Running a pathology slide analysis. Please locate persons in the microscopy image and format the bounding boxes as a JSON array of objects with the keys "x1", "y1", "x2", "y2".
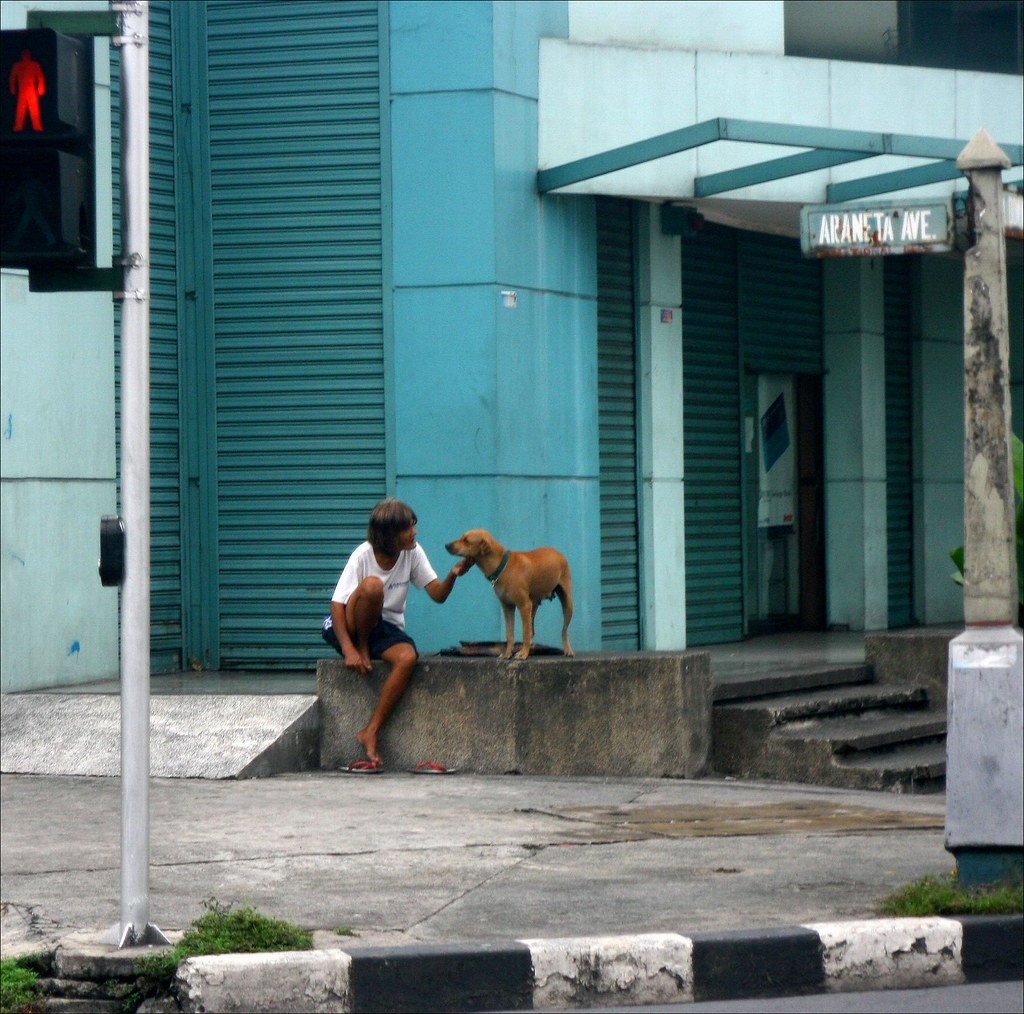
[{"x1": 322, "y1": 498, "x2": 475, "y2": 762}]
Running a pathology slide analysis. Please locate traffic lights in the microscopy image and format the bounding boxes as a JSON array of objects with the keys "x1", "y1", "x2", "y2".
[{"x1": 0, "y1": 25, "x2": 96, "y2": 268}]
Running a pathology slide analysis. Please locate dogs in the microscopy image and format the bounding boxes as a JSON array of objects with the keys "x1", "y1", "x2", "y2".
[{"x1": 445, "y1": 529, "x2": 574, "y2": 661}]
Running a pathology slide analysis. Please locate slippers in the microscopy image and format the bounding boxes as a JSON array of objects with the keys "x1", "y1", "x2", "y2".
[
  {"x1": 338, "y1": 761, "x2": 384, "y2": 773},
  {"x1": 407, "y1": 761, "x2": 456, "y2": 774}
]
